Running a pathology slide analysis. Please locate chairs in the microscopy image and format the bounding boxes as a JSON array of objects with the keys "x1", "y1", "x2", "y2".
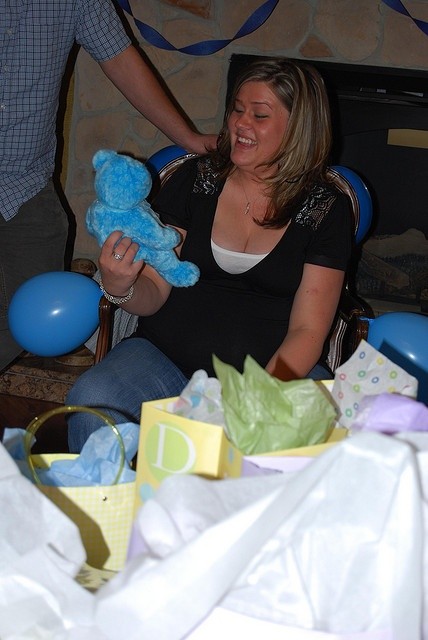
[{"x1": 93, "y1": 142, "x2": 371, "y2": 376}]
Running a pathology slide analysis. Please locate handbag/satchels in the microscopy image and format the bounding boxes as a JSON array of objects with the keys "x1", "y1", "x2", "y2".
[
  {"x1": 136, "y1": 377, "x2": 350, "y2": 512},
  {"x1": 13, "y1": 451, "x2": 138, "y2": 570}
]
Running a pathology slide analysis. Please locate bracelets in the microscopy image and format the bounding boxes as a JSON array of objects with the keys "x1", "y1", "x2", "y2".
[{"x1": 98, "y1": 277, "x2": 134, "y2": 305}]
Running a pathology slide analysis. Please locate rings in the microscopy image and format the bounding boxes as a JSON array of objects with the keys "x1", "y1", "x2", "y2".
[{"x1": 112, "y1": 251, "x2": 123, "y2": 261}]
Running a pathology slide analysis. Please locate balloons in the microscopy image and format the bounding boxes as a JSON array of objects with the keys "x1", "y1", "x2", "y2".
[
  {"x1": 358, "y1": 310, "x2": 428, "y2": 404},
  {"x1": 8, "y1": 271, "x2": 103, "y2": 357}
]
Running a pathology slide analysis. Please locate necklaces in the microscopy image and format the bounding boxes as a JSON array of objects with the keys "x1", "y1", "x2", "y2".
[{"x1": 237, "y1": 167, "x2": 272, "y2": 216}]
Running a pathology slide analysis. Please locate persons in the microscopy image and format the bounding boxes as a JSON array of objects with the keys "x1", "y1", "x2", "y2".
[
  {"x1": 0, "y1": 0, "x2": 220, "y2": 371},
  {"x1": 64, "y1": 59, "x2": 355, "y2": 454}
]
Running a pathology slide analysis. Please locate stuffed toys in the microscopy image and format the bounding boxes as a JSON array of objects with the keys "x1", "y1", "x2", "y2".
[{"x1": 85, "y1": 148, "x2": 201, "y2": 288}]
